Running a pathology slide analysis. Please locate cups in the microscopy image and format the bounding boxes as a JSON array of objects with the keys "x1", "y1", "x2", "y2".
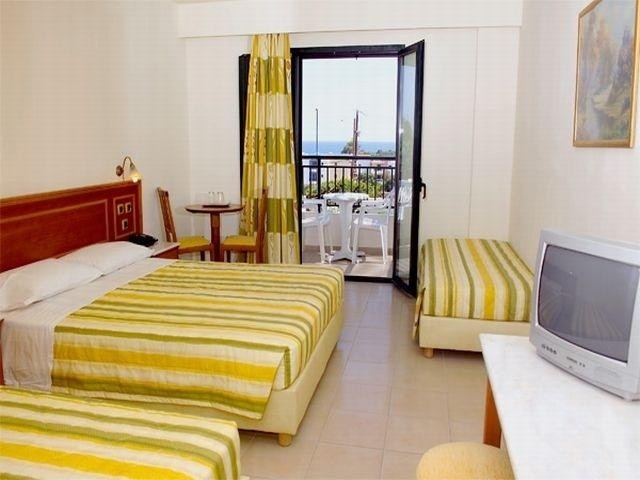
[
  {"x1": 216, "y1": 191, "x2": 224, "y2": 205},
  {"x1": 208, "y1": 191, "x2": 216, "y2": 204}
]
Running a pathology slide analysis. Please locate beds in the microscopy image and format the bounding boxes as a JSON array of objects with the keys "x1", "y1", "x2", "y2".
[
  {"x1": 0, "y1": 386, "x2": 250, "y2": 480},
  {"x1": 0, "y1": 182, "x2": 346, "y2": 445}
]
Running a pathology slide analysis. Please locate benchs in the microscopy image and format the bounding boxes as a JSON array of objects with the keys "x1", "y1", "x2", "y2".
[{"x1": 411, "y1": 237, "x2": 535, "y2": 359}]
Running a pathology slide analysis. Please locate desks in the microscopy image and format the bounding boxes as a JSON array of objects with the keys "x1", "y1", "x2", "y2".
[
  {"x1": 186, "y1": 204, "x2": 243, "y2": 261},
  {"x1": 322, "y1": 192, "x2": 369, "y2": 264},
  {"x1": 479, "y1": 334, "x2": 640, "y2": 480}
]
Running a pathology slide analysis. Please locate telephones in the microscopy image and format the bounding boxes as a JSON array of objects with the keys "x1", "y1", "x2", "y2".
[{"x1": 128, "y1": 233, "x2": 158, "y2": 247}]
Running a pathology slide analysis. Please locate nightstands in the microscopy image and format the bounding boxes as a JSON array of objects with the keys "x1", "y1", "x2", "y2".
[{"x1": 127, "y1": 234, "x2": 180, "y2": 259}]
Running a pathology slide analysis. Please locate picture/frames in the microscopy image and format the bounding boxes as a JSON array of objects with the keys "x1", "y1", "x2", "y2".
[{"x1": 573, "y1": 0, "x2": 640, "y2": 147}]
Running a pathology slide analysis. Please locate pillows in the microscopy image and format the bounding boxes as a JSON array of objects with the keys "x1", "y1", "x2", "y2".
[
  {"x1": 58, "y1": 241, "x2": 155, "y2": 276},
  {"x1": 0, "y1": 258, "x2": 104, "y2": 310}
]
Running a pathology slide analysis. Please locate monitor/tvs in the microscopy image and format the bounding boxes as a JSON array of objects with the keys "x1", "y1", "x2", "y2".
[{"x1": 529, "y1": 230, "x2": 640, "y2": 403}]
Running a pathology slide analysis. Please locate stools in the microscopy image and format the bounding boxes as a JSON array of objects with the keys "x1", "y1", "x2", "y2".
[{"x1": 416, "y1": 441, "x2": 514, "y2": 480}]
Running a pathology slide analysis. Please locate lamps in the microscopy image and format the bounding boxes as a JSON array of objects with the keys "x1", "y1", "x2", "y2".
[{"x1": 116, "y1": 156, "x2": 142, "y2": 184}]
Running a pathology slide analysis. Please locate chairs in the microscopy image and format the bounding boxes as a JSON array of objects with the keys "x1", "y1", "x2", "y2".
[
  {"x1": 299, "y1": 192, "x2": 333, "y2": 263},
  {"x1": 154, "y1": 187, "x2": 215, "y2": 261},
  {"x1": 351, "y1": 188, "x2": 395, "y2": 264},
  {"x1": 220, "y1": 189, "x2": 267, "y2": 262}
]
